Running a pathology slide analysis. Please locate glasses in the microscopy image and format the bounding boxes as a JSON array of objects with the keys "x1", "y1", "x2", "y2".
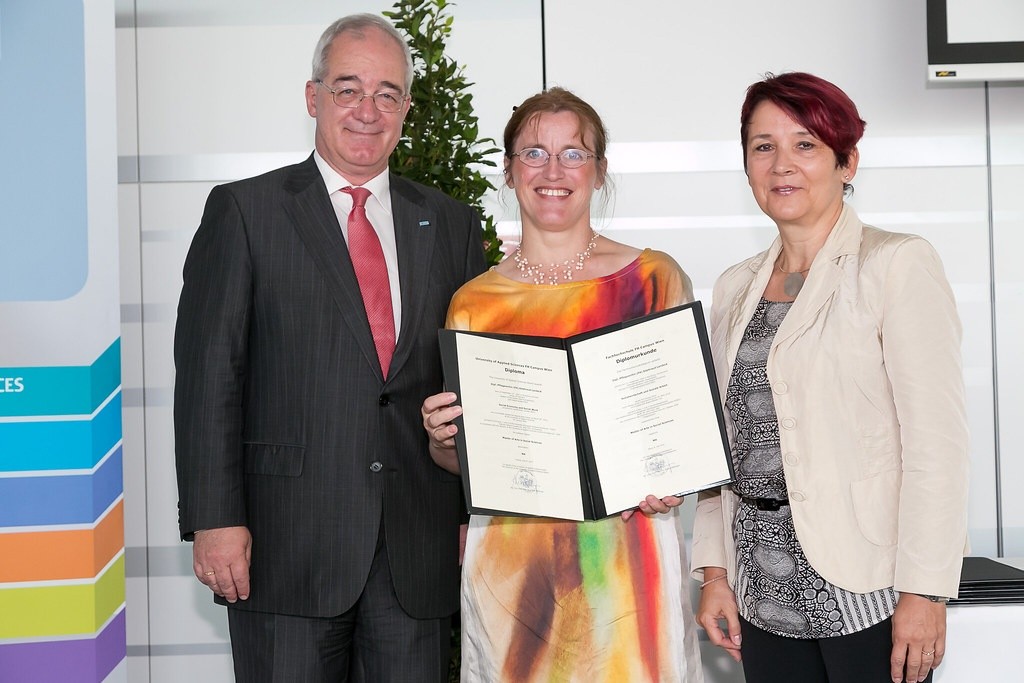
[
  {"x1": 509, "y1": 148, "x2": 599, "y2": 169},
  {"x1": 315, "y1": 79, "x2": 409, "y2": 113}
]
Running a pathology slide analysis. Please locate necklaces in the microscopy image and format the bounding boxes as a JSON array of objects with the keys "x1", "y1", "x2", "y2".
[
  {"x1": 777, "y1": 259, "x2": 810, "y2": 274},
  {"x1": 514, "y1": 228, "x2": 600, "y2": 285}
]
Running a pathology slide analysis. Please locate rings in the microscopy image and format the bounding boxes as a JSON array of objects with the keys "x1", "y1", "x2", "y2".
[
  {"x1": 203, "y1": 571, "x2": 215, "y2": 576},
  {"x1": 921, "y1": 649, "x2": 935, "y2": 656}
]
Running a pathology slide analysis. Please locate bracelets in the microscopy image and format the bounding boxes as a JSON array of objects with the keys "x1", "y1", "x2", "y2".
[{"x1": 699, "y1": 573, "x2": 727, "y2": 590}]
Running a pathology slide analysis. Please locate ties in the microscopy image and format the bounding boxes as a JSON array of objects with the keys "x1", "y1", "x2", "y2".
[{"x1": 338, "y1": 186, "x2": 396, "y2": 382}]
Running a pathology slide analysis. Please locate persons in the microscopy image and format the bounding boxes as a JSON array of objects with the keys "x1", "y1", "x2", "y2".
[
  {"x1": 690, "y1": 72, "x2": 970, "y2": 683},
  {"x1": 422, "y1": 87, "x2": 705, "y2": 683},
  {"x1": 173, "y1": 12, "x2": 489, "y2": 683}
]
